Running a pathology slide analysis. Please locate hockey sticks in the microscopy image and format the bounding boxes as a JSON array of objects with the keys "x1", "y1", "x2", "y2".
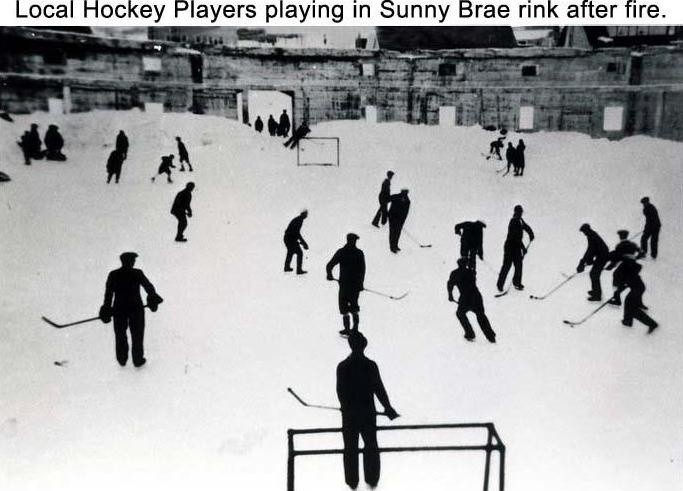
[
  {"x1": 40, "y1": 303, "x2": 149, "y2": 329},
  {"x1": 492, "y1": 241, "x2": 531, "y2": 296},
  {"x1": 529, "y1": 261, "x2": 588, "y2": 300},
  {"x1": 564, "y1": 284, "x2": 629, "y2": 326},
  {"x1": 333, "y1": 277, "x2": 409, "y2": 301},
  {"x1": 402, "y1": 226, "x2": 432, "y2": 249},
  {"x1": 286, "y1": 388, "x2": 386, "y2": 416}
]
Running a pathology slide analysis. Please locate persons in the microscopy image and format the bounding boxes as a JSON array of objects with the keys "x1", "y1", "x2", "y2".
[
  {"x1": 385, "y1": 189, "x2": 410, "y2": 252},
  {"x1": 448, "y1": 255, "x2": 496, "y2": 342},
  {"x1": 19, "y1": 122, "x2": 196, "y2": 241},
  {"x1": 336, "y1": 331, "x2": 398, "y2": 489},
  {"x1": 455, "y1": 221, "x2": 486, "y2": 273},
  {"x1": 100, "y1": 252, "x2": 163, "y2": 365},
  {"x1": 327, "y1": 234, "x2": 365, "y2": 336},
  {"x1": 497, "y1": 205, "x2": 534, "y2": 292},
  {"x1": 256, "y1": 110, "x2": 312, "y2": 148},
  {"x1": 578, "y1": 197, "x2": 660, "y2": 333},
  {"x1": 372, "y1": 171, "x2": 395, "y2": 226},
  {"x1": 487, "y1": 136, "x2": 527, "y2": 176},
  {"x1": 284, "y1": 210, "x2": 308, "y2": 274}
]
